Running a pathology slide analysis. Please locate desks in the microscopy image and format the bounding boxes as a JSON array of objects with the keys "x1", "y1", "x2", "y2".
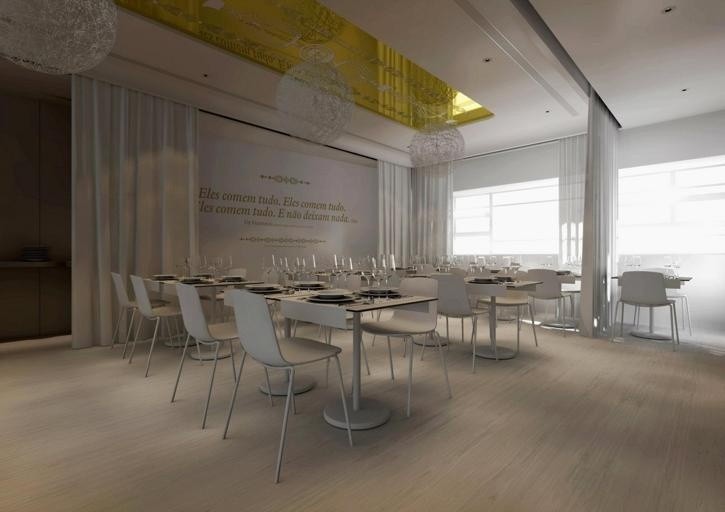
[
  {"x1": 611, "y1": 275, "x2": 691, "y2": 341},
  {"x1": 257, "y1": 290, "x2": 439, "y2": 431}
]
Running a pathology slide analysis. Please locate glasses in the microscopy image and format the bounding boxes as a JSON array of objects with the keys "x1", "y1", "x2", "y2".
[
  {"x1": 0, "y1": 0, "x2": 117, "y2": 75},
  {"x1": 276, "y1": 23, "x2": 354, "y2": 146},
  {"x1": 409, "y1": 106, "x2": 466, "y2": 170}
]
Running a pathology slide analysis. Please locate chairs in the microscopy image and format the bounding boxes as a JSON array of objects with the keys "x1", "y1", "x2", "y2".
[
  {"x1": 656, "y1": 270, "x2": 691, "y2": 332},
  {"x1": 107, "y1": 255, "x2": 575, "y2": 375},
  {"x1": 610, "y1": 271, "x2": 681, "y2": 351},
  {"x1": 360, "y1": 277, "x2": 453, "y2": 418},
  {"x1": 170, "y1": 282, "x2": 274, "y2": 429},
  {"x1": 222, "y1": 289, "x2": 354, "y2": 480},
  {"x1": 614, "y1": 272, "x2": 643, "y2": 330}
]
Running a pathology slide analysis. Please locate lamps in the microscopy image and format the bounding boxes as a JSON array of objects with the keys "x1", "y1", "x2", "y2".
[
  {"x1": 276, "y1": 23, "x2": 354, "y2": 146},
  {"x1": 0, "y1": 0, "x2": 117, "y2": 75},
  {"x1": 409, "y1": 106, "x2": 466, "y2": 170}
]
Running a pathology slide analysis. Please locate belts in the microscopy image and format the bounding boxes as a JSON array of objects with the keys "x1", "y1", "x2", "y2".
[
  {"x1": 321, "y1": 251, "x2": 354, "y2": 289},
  {"x1": 622, "y1": 255, "x2": 641, "y2": 270},
  {"x1": 407, "y1": 254, "x2": 460, "y2": 274},
  {"x1": 262, "y1": 253, "x2": 317, "y2": 294},
  {"x1": 543, "y1": 254, "x2": 581, "y2": 273},
  {"x1": 190, "y1": 254, "x2": 234, "y2": 281},
  {"x1": 484, "y1": 254, "x2": 523, "y2": 285},
  {"x1": 662, "y1": 253, "x2": 682, "y2": 278},
  {"x1": 361, "y1": 251, "x2": 397, "y2": 304}
]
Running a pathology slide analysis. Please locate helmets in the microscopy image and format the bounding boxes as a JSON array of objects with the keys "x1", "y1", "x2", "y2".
[
  {"x1": 469, "y1": 277, "x2": 500, "y2": 285},
  {"x1": 151, "y1": 272, "x2": 245, "y2": 284},
  {"x1": 292, "y1": 278, "x2": 331, "y2": 290},
  {"x1": 244, "y1": 283, "x2": 285, "y2": 293},
  {"x1": 358, "y1": 285, "x2": 400, "y2": 297},
  {"x1": 305, "y1": 289, "x2": 356, "y2": 303}
]
